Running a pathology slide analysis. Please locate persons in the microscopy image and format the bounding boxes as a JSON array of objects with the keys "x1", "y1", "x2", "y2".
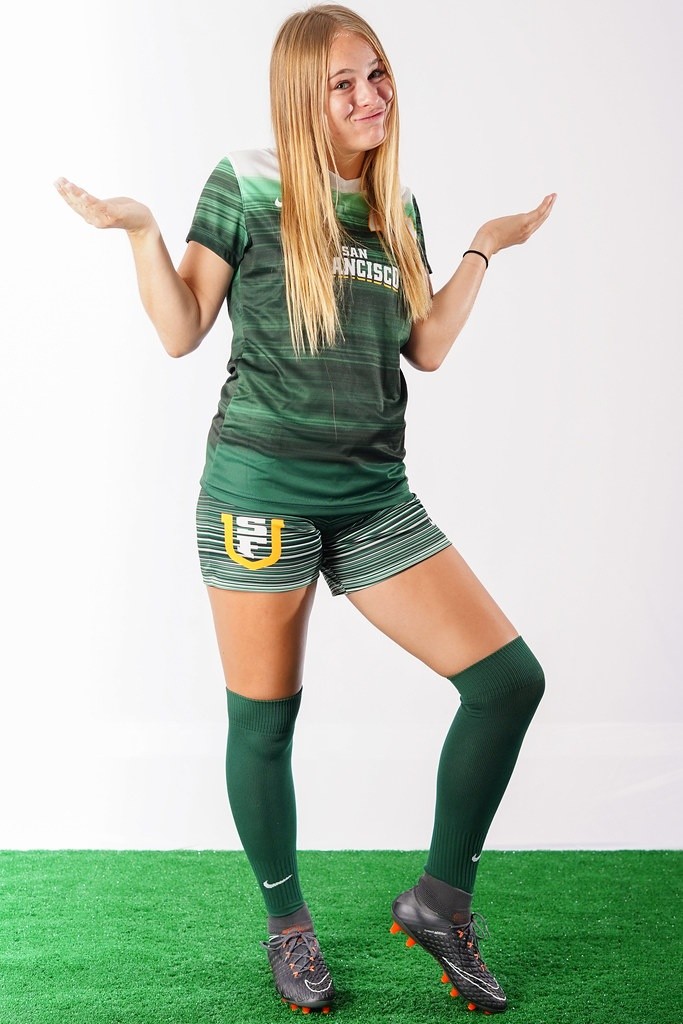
[{"x1": 52, "y1": 5, "x2": 558, "y2": 1014}]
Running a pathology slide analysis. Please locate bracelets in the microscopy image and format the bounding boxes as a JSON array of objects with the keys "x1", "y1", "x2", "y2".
[{"x1": 463, "y1": 250, "x2": 488, "y2": 269}]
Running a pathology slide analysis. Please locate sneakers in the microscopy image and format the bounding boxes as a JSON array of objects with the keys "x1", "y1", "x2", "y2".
[
  {"x1": 266, "y1": 901, "x2": 338, "y2": 1015},
  {"x1": 389, "y1": 886, "x2": 508, "y2": 1012}
]
